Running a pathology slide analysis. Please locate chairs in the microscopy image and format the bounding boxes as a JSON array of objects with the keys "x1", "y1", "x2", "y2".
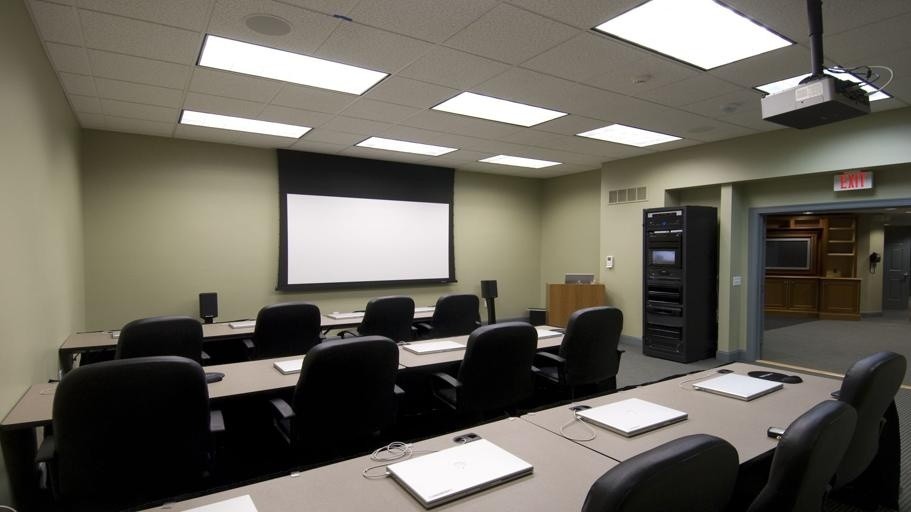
[
  {"x1": 337, "y1": 295, "x2": 418, "y2": 344},
  {"x1": 582, "y1": 434, "x2": 739, "y2": 512},
  {"x1": 115, "y1": 315, "x2": 210, "y2": 366},
  {"x1": 415, "y1": 294, "x2": 481, "y2": 340},
  {"x1": 748, "y1": 400, "x2": 857, "y2": 512},
  {"x1": 537, "y1": 306, "x2": 625, "y2": 399},
  {"x1": 269, "y1": 336, "x2": 405, "y2": 463},
  {"x1": 433, "y1": 322, "x2": 538, "y2": 421},
  {"x1": 823, "y1": 352, "x2": 906, "y2": 512},
  {"x1": 243, "y1": 302, "x2": 326, "y2": 361},
  {"x1": 36, "y1": 356, "x2": 225, "y2": 512}
]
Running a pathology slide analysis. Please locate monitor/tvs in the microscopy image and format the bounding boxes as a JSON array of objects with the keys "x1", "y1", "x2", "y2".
[{"x1": 764, "y1": 238, "x2": 809, "y2": 269}]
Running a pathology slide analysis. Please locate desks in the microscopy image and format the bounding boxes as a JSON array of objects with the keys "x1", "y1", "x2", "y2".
[
  {"x1": 521, "y1": 361, "x2": 842, "y2": 465},
  {"x1": 397, "y1": 327, "x2": 564, "y2": 371},
  {"x1": 0, "y1": 354, "x2": 406, "y2": 512},
  {"x1": 140, "y1": 417, "x2": 620, "y2": 512},
  {"x1": 321, "y1": 306, "x2": 435, "y2": 330},
  {"x1": 60, "y1": 320, "x2": 256, "y2": 371}
]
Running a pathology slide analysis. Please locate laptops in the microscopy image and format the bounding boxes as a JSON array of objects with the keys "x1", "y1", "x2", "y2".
[
  {"x1": 416, "y1": 307, "x2": 436, "y2": 312},
  {"x1": 231, "y1": 319, "x2": 257, "y2": 329},
  {"x1": 111, "y1": 331, "x2": 119, "y2": 337},
  {"x1": 327, "y1": 310, "x2": 367, "y2": 321},
  {"x1": 536, "y1": 327, "x2": 564, "y2": 338},
  {"x1": 565, "y1": 273, "x2": 595, "y2": 284},
  {"x1": 385, "y1": 437, "x2": 535, "y2": 509},
  {"x1": 275, "y1": 356, "x2": 304, "y2": 376},
  {"x1": 405, "y1": 338, "x2": 469, "y2": 355},
  {"x1": 576, "y1": 398, "x2": 685, "y2": 438},
  {"x1": 691, "y1": 371, "x2": 784, "y2": 401}
]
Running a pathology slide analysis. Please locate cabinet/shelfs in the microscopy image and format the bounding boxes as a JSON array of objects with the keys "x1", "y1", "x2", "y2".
[
  {"x1": 764, "y1": 275, "x2": 790, "y2": 317},
  {"x1": 824, "y1": 214, "x2": 856, "y2": 256},
  {"x1": 766, "y1": 215, "x2": 824, "y2": 229},
  {"x1": 546, "y1": 282, "x2": 605, "y2": 328},
  {"x1": 818, "y1": 278, "x2": 862, "y2": 321},
  {"x1": 790, "y1": 276, "x2": 818, "y2": 317}
]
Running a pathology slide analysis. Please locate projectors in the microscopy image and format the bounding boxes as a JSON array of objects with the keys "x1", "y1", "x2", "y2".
[{"x1": 763, "y1": 76, "x2": 870, "y2": 130}]
